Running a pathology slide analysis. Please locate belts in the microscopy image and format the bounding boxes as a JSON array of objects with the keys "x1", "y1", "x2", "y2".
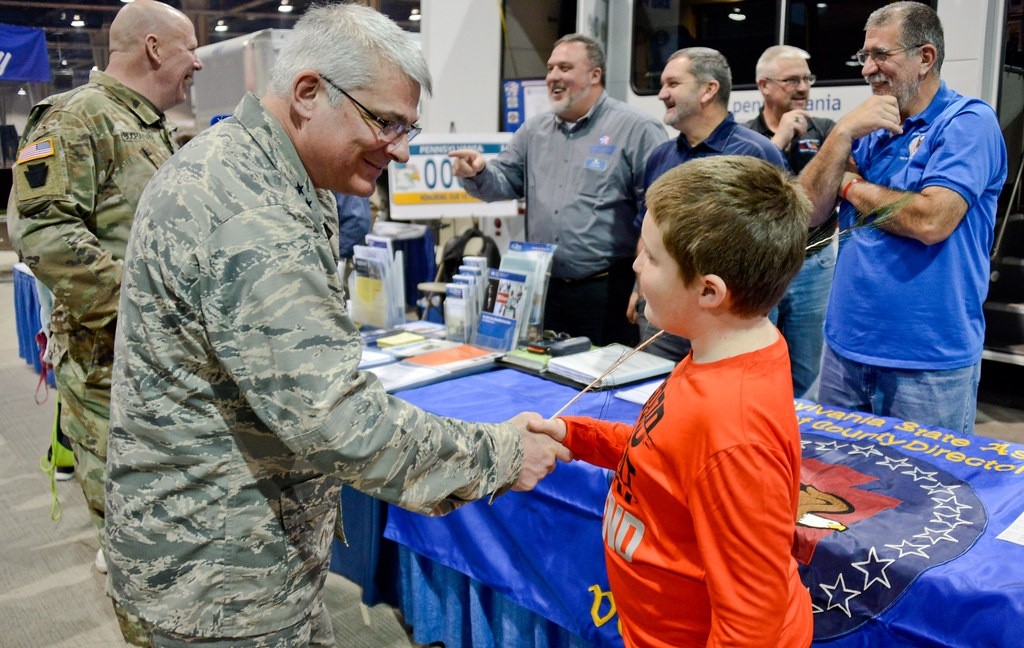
[
  {"x1": 804, "y1": 232, "x2": 836, "y2": 255},
  {"x1": 551, "y1": 263, "x2": 612, "y2": 284}
]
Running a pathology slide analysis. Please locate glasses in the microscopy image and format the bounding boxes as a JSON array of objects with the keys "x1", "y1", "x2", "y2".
[
  {"x1": 767, "y1": 75, "x2": 820, "y2": 89},
  {"x1": 316, "y1": 73, "x2": 422, "y2": 143},
  {"x1": 853, "y1": 44, "x2": 918, "y2": 66}
]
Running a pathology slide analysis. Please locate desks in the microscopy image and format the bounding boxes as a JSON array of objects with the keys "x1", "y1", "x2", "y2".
[
  {"x1": 13, "y1": 260, "x2": 56, "y2": 390},
  {"x1": 329, "y1": 320, "x2": 1024, "y2": 648}
]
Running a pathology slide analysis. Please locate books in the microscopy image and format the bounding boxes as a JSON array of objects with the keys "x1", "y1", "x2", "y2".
[
  {"x1": 446, "y1": 256, "x2": 487, "y2": 342},
  {"x1": 502, "y1": 342, "x2": 599, "y2": 369},
  {"x1": 548, "y1": 343, "x2": 676, "y2": 387}
]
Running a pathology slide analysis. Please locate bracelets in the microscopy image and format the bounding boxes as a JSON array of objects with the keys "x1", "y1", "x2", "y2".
[{"x1": 842, "y1": 178, "x2": 864, "y2": 200}]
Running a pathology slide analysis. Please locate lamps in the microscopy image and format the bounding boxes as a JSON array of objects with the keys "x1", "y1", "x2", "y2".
[
  {"x1": 70, "y1": 8, "x2": 85, "y2": 27},
  {"x1": 728, "y1": 7, "x2": 746, "y2": 22},
  {"x1": 215, "y1": 19, "x2": 229, "y2": 32},
  {"x1": 408, "y1": 3, "x2": 421, "y2": 21},
  {"x1": 278, "y1": 0, "x2": 294, "y2": 13}
]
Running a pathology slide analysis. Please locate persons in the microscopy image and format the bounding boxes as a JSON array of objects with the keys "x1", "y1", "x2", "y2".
[
  {"x1": 104, "y1": 3, "x2": 573, "y2": 648},
  {"x1": 447, "y1": 34, "x2": 670, "y2": 347},
  {"x1": 633, "y1": 47, "x2": 790, "y2": 367},
  {"x1": 528, "y1": 155, "x2": 815, "y2": 648},
  {"x1": 7, "y1": 0, "x2": 203, "y2": 576},
  {"x1": 798, "y1": 1, "x2": 1008, "y2": 434},
  {"x1": 333, "y1": 191, "x2": 371, "y2": 256},
  {"x1": 742, "y1": 45, "x2": 837, "y2": 398}
]
